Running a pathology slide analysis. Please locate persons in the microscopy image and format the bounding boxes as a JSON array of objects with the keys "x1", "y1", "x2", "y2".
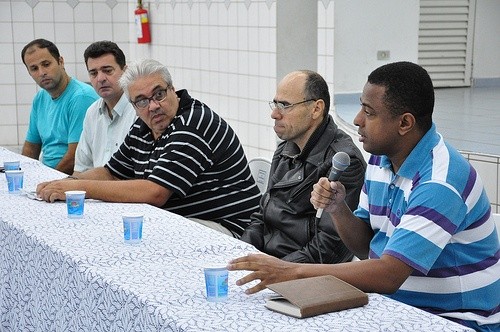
[
  {"x1": 227, "y1": 61, "x2": 500, "y2": 332},
  {"x1": 240, "y1": 70, "x2": 368, "y2": 266},
  {"x1": 36, "y1": 59, "x2": 263, "y2": 239},
  {"x1": 71, "y1": 40, "x2": 139, "y2": 177},
  {"x1": 20, "y1": 39, "x2": 102, "y2": 176}
]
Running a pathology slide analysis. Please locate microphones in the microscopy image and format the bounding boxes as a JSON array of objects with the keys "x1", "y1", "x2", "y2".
[{"x1": 314, "y1": 152, "x2": 350, "y2": 218}]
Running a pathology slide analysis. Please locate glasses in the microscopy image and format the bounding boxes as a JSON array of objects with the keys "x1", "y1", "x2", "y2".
[
  {"x1": 129, "y1": 85, "x2": 171, "y2": 110},
  {"x1": 269, "y1": 99, "x2": 317, "y2": 113}
]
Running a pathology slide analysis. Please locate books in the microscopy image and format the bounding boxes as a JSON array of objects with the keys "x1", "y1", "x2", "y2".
[{"x1": 264, "y1": 274, "x2": 369, "y2": 319}]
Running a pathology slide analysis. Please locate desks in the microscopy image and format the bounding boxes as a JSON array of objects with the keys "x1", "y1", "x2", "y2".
[{"x1": 0, "y1": 146, "x2": 476, "y2": 331}]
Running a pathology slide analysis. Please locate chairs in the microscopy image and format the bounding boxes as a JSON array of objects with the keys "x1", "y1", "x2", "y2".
[{"x1": 247, "y1": 158, "x2": 272, "y2": 194}]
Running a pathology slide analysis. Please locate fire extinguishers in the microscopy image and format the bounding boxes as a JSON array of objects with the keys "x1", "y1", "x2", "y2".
[{"x1": 135, "y1": 0, "x2": 151, "y2": 44}]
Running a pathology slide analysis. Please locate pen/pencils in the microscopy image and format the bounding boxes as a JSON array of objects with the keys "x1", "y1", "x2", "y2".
[{"x1": 1, "y1": 168, "x2": 21, "y2": 173}]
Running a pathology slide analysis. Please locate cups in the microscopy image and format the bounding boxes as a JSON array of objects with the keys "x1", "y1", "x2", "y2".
[
  {"x1": 64, "y1": 190, "x2": 86, "y2": 219},
  {"x1": 203, "y1": 263, "x2": 229, "y2": 302},
  {"x1": 5, "y1": 169, "x2": 24, "y2": 193},
  {"x1": 3, "y1": 160, "x2": 21, "y2": 169},
  {"x1": 121, "y1": 213, "x2": 144, "y2": 243}
]
